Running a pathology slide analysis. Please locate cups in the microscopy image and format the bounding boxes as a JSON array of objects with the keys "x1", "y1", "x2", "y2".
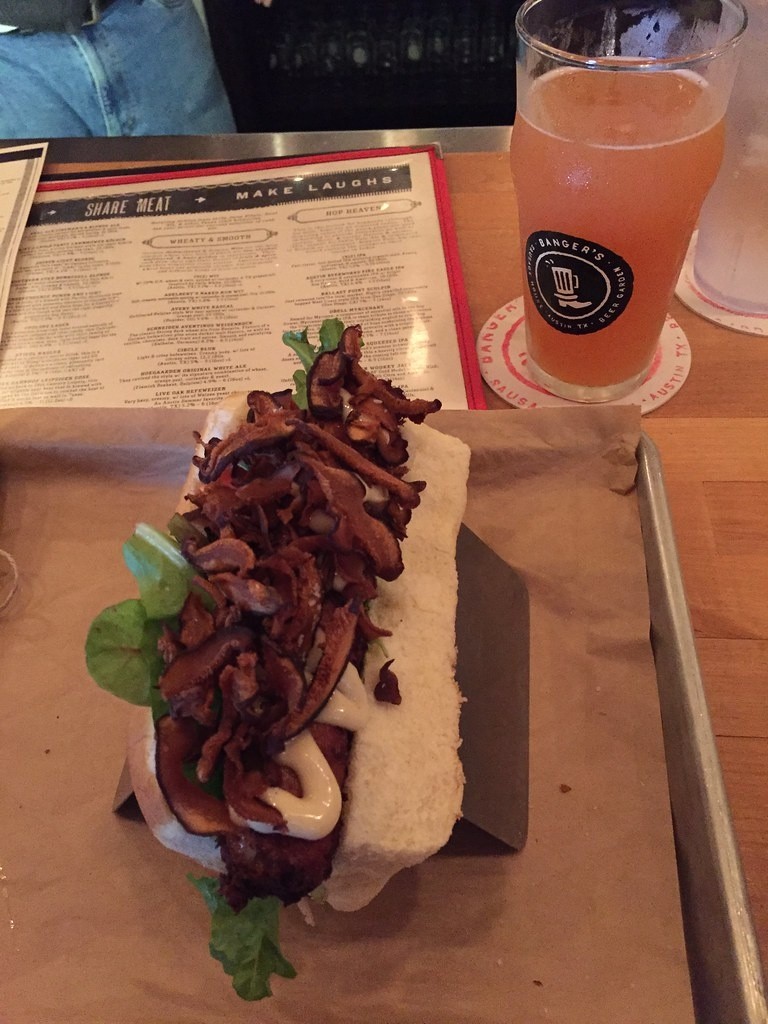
[
  {"x1": 691, "y1": 1, "x2": 768, "y2": 319},
  {"x1": 510, "y1": 1, "x2": 750, "y2": 400}
]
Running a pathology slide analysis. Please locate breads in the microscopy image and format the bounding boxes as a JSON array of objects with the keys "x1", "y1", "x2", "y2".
[{"x1": 129, "y1": 394, "x2": 470, "y2": 912}]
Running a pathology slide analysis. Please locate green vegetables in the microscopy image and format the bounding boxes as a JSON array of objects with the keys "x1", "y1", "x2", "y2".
[
  {"x1": 82, "y1": 513, "x2": 300, "y2": 1003},
  {"x1": 281, "y1": 313, "x2": 365, "y2": 409}
]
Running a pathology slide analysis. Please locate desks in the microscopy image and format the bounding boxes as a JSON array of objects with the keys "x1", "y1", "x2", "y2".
[{"x1": 2, "y1": 124, "x2": 767, "y2": 989}]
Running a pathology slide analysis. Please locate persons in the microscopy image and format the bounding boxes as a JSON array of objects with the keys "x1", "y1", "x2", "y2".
[{"x1": 0, "y1": 0, "x2": 236, "y2": 139}]
